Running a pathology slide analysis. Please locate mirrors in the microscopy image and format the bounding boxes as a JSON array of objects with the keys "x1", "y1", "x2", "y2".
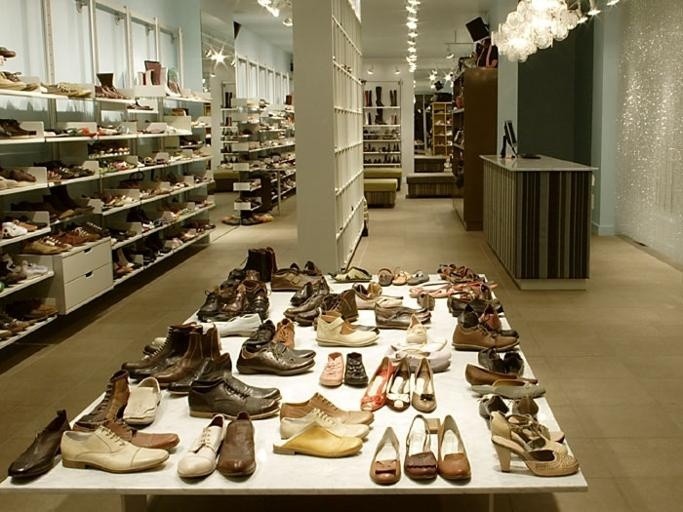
[{"x1": 198, "y1": 9, "x2": 241, "y2": 242}]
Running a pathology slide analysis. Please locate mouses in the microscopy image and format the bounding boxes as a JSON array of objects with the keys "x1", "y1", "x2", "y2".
[{"x1": 527, "y1": 153, "x2": 536, "y2": 157}]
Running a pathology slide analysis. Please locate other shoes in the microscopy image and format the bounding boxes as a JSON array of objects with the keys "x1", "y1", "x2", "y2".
[{"x1": 2, "y1": 59, "x2": 212, "y2": 357}]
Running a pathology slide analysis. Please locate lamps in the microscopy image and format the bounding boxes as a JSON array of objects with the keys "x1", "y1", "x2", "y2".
[
  {"x1": 404, "y1": 1, "x2": 419, "y2": 23},
  {"x1": 487, "y1": 1, "x2": 619, "y2": 65}
]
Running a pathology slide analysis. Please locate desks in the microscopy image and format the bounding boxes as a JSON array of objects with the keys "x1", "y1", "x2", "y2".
[{"x1": 2, "y1": 269, "x2": 589, "y2": 508}]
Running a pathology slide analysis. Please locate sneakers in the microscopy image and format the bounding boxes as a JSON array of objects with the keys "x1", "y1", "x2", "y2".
[{"x1": 363, "y1": 129, "x2": 401, "y2": 167}]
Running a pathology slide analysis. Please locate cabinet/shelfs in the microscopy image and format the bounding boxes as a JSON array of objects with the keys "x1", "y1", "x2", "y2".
[
  {"x1": 362, "y1": 103, "x2": 401, "y2": 166},
  {"x1": 291, "y1": 0, "x2": 365, "y2": 274},
  {"x1": 449, "y1": 66, "x2": 497, "y2": 230},
  {"x1": 1, "y1": 88, "x2": 212, "y2": 349},
  {"x1": 216, "y1": 106, "x2": 237, "y2": 168},
  {"x1": 238, "y1": 106, "x2": 295, "y2": 219},
  {"x1": 428, "y1": 101, "x2": 452, "y2": 157}
]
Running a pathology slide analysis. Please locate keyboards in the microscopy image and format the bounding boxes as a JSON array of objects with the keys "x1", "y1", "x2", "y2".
[{"x1": 523, "y1": 156, "x2": 541, "y2": 159}]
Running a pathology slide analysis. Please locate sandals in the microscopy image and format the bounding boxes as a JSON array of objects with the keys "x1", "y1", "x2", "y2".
[
  {"x1": 478, "y1": 394, "x2": 530, "y2": 426},
  {"x1": 488, "y1": 410, "x2": 565, "y2": 444},
  {"x1": 472, "y1": 377, "x2": 546, "y2": 400},
  {"x1": 361, "y1": 356, "x2": 394, "y2": 411}
]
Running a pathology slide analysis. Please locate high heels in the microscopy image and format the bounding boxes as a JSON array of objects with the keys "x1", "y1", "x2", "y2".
[{"x1": 491, "y1": 435, "x2": 580, "y2": 477}]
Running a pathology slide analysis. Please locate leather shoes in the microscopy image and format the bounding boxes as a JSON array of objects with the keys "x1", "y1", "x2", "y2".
[
  {"x1": 466, "y1": 364, "x2": 539, "y2": 385},
  {"x1": 387, "y1": 358, "x2": 413, "y2": 410},
  {"x1": 188, "y1": 371, "x2": 282, "y2": 418},
  {"x1": 371, "y1": 425, "x2": 401, "y2": 486},
  {"x1": 409, "y1": 357, "x2": 437, "y2": 411},
  {"x1": 272, "y1": 392, "x2": 374, "y2": 460},
  {"x1": 8, "y1": 367, "x2": 179, "y2": 480},
  {"x1": 315, "y1": 260, "x2": 526, "y2": 377},
  {"x1": 219, "y1": 91, "x2": 295, "y2": 226},
  {"x1": 404, "y1": 414, "x2": 436, "y2": 481},
  {"x1": 437, "y1": 414, "x2": 470, "y2": 480},
  {"x1": 319, "y1": 352, "x2": 344, "y2": 386},
  {"x1": 120, "y1": 247, "x2": 360, "y2": 396},
  {"x1": 346, "y1": 352, "x2": 366, "y2": 386},
  {"x1": 175, "y1": 412, "x2": 257, "y2": 479}
]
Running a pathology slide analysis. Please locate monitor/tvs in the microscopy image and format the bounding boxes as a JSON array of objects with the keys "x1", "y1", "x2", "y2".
[
  {"x1": 465, "y1": 16, "x2": 490, "y2": 42},
  {"x1": 500, "y1": 120, "x2": 517, "y2": 159}
]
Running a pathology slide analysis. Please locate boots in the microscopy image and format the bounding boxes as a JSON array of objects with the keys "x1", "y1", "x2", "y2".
[{"x1": 364, "y1": 86, "x2": 398, "y2": 125}]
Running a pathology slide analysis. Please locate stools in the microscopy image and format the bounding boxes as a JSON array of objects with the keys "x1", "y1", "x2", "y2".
[
  {"x1": 363, "y1": 178, "x2": 398, "y2": 207},
  {"x1": 363, "y1": 167, "x2": 401, "y2": 190}
]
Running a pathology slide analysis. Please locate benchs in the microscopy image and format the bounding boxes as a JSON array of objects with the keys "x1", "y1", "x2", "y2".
[
  {"x1": 414, "y1": 155, "x2": 446, "y2": 172},
  {"x1": 405, "y1": 173, "x2": 452, "y2": 198}
]
